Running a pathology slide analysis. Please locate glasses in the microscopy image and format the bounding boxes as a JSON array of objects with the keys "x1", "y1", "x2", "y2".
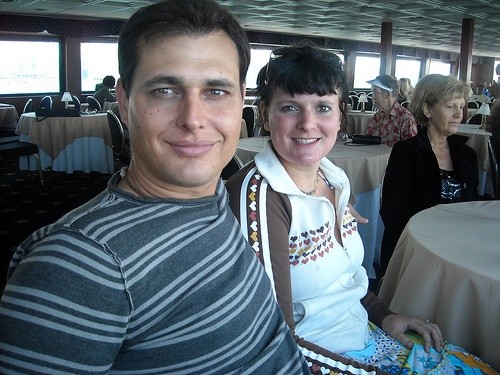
[{"x1": 263, "y1": 44, "x2": 350, "y2": 85}]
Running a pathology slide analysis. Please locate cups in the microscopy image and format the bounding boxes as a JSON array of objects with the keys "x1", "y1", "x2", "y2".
[{"x1": 81, "y1": 103, "x2": 89, "y2": 112}]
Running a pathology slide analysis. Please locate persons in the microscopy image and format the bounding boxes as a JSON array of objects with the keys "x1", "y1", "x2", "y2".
[
  {"x1": 91, "y1": 76, "x2": 117, "y2": 107},
  {"x1": 395, "y1": 77, "x2": 413, "y2": 106},
  {"x1": 0, "y1": 0, "x2": 369, "y2": 375},
  {"x1": 467, "y1": 64, "x2": 500, "y2": 173},
  {"x1": 225, "y1": 45, "x2": 500, "y2": 375},
  {"x1": 362, "y1": 74, "x2": 416, "y2": 147},
  {"x1": 376, "y1": 74, "x2": 484, "y2": 297}
]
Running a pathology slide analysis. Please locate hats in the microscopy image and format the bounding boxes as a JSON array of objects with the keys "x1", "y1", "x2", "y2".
[{"x1": 365, "y1": 79, "x2": 393, "y2": 93}]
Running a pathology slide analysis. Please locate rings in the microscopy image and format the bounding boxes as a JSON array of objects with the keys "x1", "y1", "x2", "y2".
[{"x1": 424, "y1": 320, "x2": 431, "y2": 323}]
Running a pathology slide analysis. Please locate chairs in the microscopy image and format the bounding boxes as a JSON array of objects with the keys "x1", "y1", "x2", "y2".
[{"x1": 0, "y1": 87, "x2": 500, "y2": 298}]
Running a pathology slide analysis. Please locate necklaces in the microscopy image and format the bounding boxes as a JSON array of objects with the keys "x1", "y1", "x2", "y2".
[
  {"x1": 120, "y1": 166, "x2": 155, "y2": 199},
  {"x1": 299, "y1": 172, "x2": 319, "y2": 196}
]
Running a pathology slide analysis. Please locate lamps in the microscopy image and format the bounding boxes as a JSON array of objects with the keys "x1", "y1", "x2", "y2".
[
  {"x1": 61, "y1": 91, "x2": 73, "y2": 110},
  {"x1": 357, "y1": 94, "x2": 369, "y2": 113},
  {"x1": 477, "y1": 103, "x2": 491, "y2": 129}
]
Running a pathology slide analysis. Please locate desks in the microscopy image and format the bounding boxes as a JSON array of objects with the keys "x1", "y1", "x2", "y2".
[
  {"x1": 376, "y1": 200, "x2": 500, "y2": 373},
  {"x1": 14, "y1": 111, "x2": 114, "y2": 177},
  {"x1": 466, "y1": 108, "x2": 479, "y2": 120},
  {"x1": 235, "y1": 135, "x2": 392, "y2": 280},
  {"x1": 0, "y1": 103, "x2": 19, "y2": 129},
  {"x1": 242, "y1": 104, "x2": 262, "y2": 137},
  {"x1": 244, "y1": 95, "x2": 261, "y2": 105},
  {"x1": 103, "y1": 100, "x2": 121, "y2": 120},
  {"x1": 341, "y1": 109, "x2": 378, "y2": 137},
  {"x1": 446, "y1": 124, "x2": 498, "y2": 199}
]
